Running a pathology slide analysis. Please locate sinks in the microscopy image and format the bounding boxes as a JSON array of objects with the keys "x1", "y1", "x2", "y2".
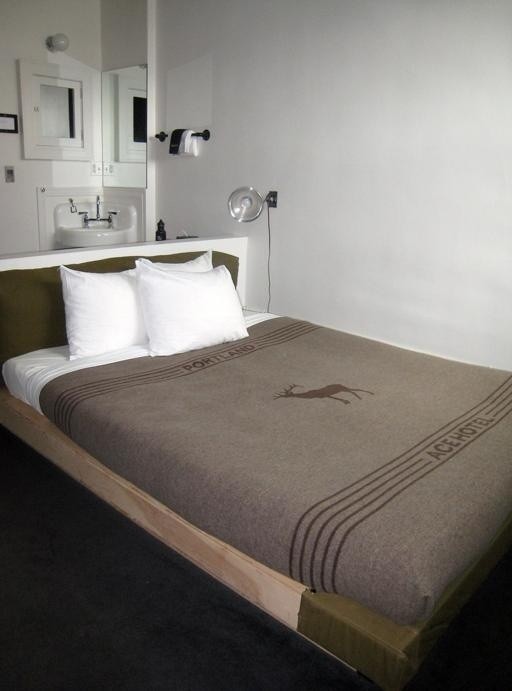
[{"x1": 59, "y1": 227, "x2": 125, "y2": 247}]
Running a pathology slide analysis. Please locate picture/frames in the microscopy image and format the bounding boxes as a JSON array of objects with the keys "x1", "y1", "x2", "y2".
[{"x1": 0, "y1": 114, "x2": 18, "y2": 133}]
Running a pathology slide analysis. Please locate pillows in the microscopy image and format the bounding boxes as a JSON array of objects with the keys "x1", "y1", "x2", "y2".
[
  {"x1": 135, "y1": 250, "x2": 213, "y2": 357},
  {"x1": 58, "y1": 263, "x2": 148, "y2": 364},
  {"x1": 135, "y1": 260, "x2": 250, "y2": 357}
]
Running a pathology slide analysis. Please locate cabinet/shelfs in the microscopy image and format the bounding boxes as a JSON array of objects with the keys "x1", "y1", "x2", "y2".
[{"x1": 18, "y1": 59, "x2": 94, "y2": 162}]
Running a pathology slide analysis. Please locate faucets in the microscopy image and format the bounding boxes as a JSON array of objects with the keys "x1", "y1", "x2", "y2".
[{"x1": 96, "y1": 195, "x2": 100, "y2": 220}]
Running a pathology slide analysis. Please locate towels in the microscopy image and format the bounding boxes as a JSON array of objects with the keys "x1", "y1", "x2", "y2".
[{"x1": 169, "y1": 128, "x2": 198, "y2": 158}]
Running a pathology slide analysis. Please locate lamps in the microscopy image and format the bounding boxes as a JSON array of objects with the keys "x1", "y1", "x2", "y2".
[
  {"x1": 46, "y1": 32, "x2": 69, "y2": 53},
  {"x1": 226, "y1": 187, "x2": 277, "y2": 223}
]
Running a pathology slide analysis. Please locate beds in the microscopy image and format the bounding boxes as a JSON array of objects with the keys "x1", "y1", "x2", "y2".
[{"x1": 0, "y1": 234, "x2": 511, "y2": 691}]
